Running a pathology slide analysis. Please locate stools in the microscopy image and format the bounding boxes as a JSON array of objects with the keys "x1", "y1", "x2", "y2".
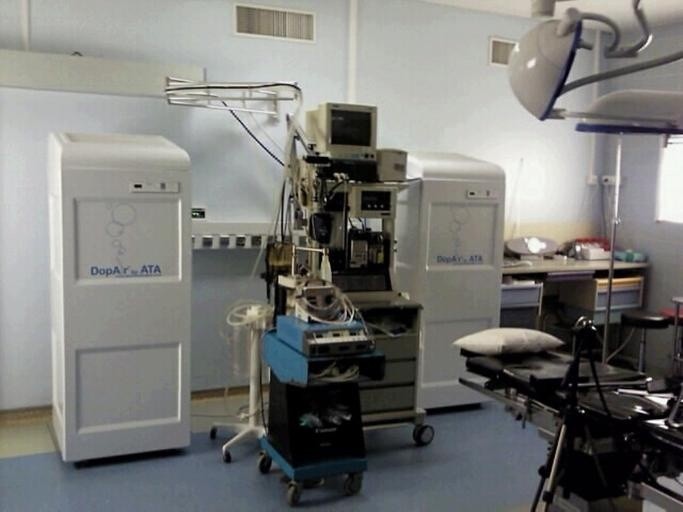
[{"x1": 618, "y1": 305, "x2": 683, "y2": 373}]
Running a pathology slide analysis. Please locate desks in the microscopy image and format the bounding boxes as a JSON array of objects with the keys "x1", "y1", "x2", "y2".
[{"x1": 501, "y1": 252, "x2": 650, "y2": 374}]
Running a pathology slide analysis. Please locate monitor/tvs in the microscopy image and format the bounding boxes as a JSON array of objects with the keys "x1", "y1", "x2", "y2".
[{"x1": 304, "y1": 101, "x2": 378, "y2": 162}]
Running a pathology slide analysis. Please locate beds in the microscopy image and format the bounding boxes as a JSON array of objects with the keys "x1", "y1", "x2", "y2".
[{"x1": 458, "y1": 341, "x2": 682, "y2": 511}]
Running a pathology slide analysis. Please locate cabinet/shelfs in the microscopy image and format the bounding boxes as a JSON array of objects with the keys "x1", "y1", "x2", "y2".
[{"x1": 351, "y1": 295, "x2": 435, "y2": 448}]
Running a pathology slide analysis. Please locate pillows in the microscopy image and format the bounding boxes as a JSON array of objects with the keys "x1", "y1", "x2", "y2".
[{"x1": 452, "y1": 326, "x2": 568, "y2": 357}]
